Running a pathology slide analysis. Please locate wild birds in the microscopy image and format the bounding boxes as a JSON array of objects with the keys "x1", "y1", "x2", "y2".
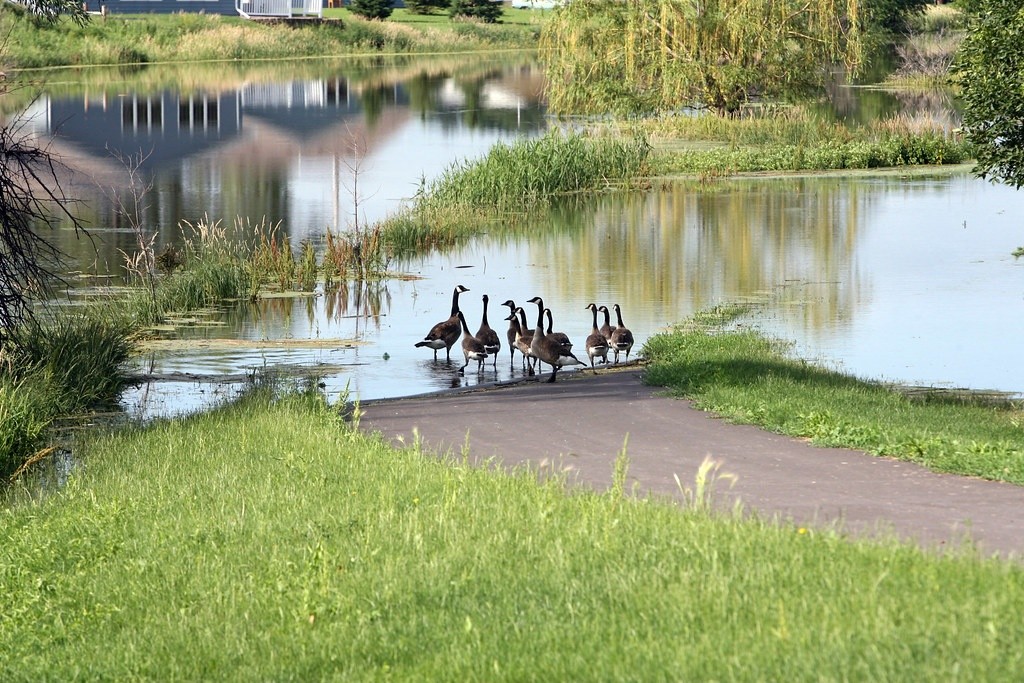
[
  {"x1": 543, "y1": 309, "x2": 572, "y2": 352},
  {"x1": 475, "y1": 295, "x2": 499, "y2": 366},
  {"x1": 500, "y1": 300, "x2": 545, "y2": 372},
  {"x1": 415, "y1": 286, "x2": 469, "y2": 364},
  {"x1": 597, "y1": 306, "x2": 617, "y2": 349},
  {"x1": 585, "y1": 303, "x2": 608, "y2": 374},
  {"x1": 527, "y1": 297, "x2": 586, "y2": 382},
  {"x1": 611, "y1": 304, "x2": 634, "y2": 365},
  {"x1": 455, "y1": 311, "x2": 488, "y2": 373}
]
